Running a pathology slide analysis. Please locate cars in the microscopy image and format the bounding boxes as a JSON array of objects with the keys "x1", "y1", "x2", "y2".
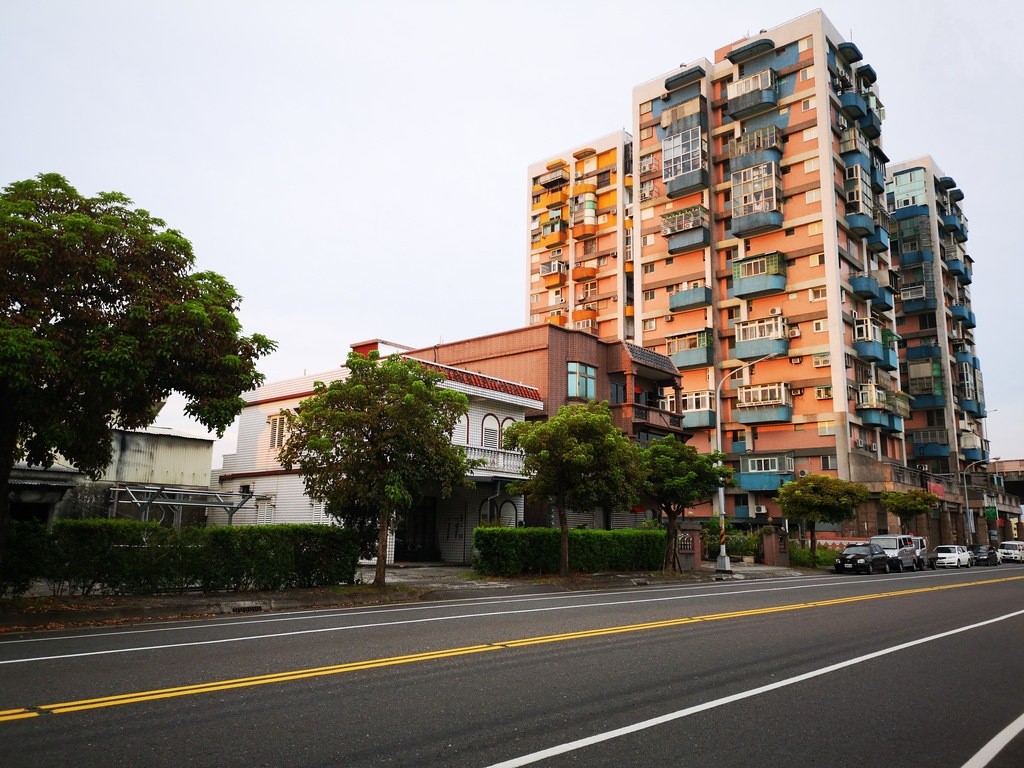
[
  {"x1": 966, "y1": 544, "x2": 998, "y2": 566},
  {"x1": 992, "y1": 546, "x2": 1004, "y2": 565},
  {"x1": 833, "y1": 542, "x2": 891, "y2": 575},
  {"x1": 932, "y1": 544, "x2": 970, "y2": 569}
]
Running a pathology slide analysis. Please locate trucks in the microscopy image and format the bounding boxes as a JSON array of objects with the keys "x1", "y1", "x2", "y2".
[{"x1": 913, "y1": 536, "x2": 938, "y2": 571}]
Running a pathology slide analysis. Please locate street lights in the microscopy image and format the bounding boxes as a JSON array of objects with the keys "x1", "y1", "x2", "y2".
[
  {"x1": 964, "y1": 456, "x2": 1001, "y2": 544},
  {"x1": 713, "y1": 352, "x2": 782, "y2": 572}
]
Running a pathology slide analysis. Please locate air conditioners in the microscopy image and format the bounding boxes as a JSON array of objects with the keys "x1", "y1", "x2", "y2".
[
  {"x1": 799, "y1": 469, "x2": 809, "y2": 478},
  {"x1": 754, "y1": 505, "x2": 767, "y2": 514},
  {"x1": 610, "y1": 209, "x2": 617, "y2": 214},
  {"x1": 664, "y1": 315, "x2": 671, "y2": 321},
  {"x1": 792, "y1": 389, "x2": 801, "y2": 395},
  {"x1": 851, "y1": 309, "x2": 857, "y2": 319},
  {"x1": 611, "y1": 167, "x2": 616, "y2": 173},
  {"x1": 769, "y1": 308, "x2": 781, "y2": 315},
  {"x1": 610, "y1": 251, "x2": 617, "y2": 256},
  {"x1": 685, "y1": 222, "x2": 693, "y2": 229},
  {"x1": 917, "y1": 464, "x2": 928, "y2": 471},
  {"x1": 641, "y1": 164, "x2": 650, "y2": 173},
  {"x1": 789, "y1": 330, "x2": 800, "y2": 338},
  {"x1": 560, "y1": 298, "x2": 566, "y2": 304},
  {"x1": 833, "y1": 78, "x2": 842, "y2": 89},
  {"x1": 839, "y1": 116, "x2": 847, "y2": 129},
  {"x1": 661, "y1": 228, "x2": 672, "y2": 236},
  {"x1": 841, "y1": 70, "x2": 849, "y2": 83},
  {"x1": 640, "y1": 191, "x2": 650, "y2": 199},
  {"x1": 792, "y1": 358, "x2": 800, "y2": 364},
  {"x1": 575, "y1": 172, "x2": 583, "y2": 179},
  {"x1": 856, "y1": 438, "x2": 864, "y2": 448},
  {"x1": 611, "y1": 296, "x2": 618, "y2": 301},
  {"x1": 577, "y1": 295, "x2": 584, "y2": 300},
  {"x1": 575, "y1": 263, "x2": 581, "y2": 267},
  {"x1": 871, "y1": 443, "x2": 877, "y2": 451}
]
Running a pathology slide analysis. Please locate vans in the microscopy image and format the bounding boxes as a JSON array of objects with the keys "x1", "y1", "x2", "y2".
[
  {"x1": 868, "y1": 534, "x2": 918, "y2": 573},
  {"x1": 998, "y1": 541, "x2": 1024, "y2": 564}
]
